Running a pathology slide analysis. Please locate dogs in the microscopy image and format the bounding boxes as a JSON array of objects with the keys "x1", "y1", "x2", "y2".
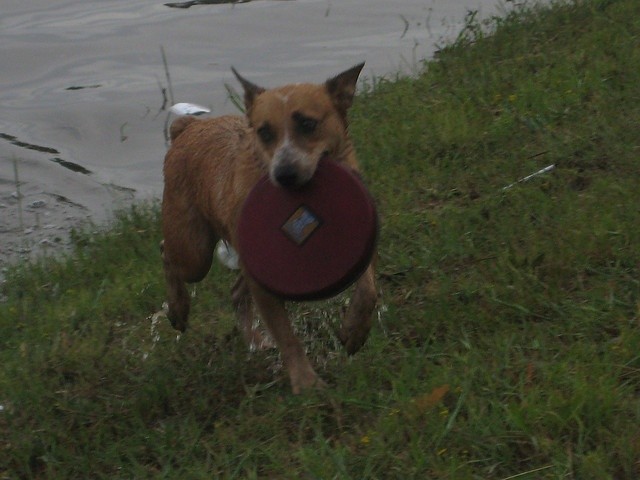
[{"x1": 160, "y1": 60, "x2": 381, "y2": 399}]
